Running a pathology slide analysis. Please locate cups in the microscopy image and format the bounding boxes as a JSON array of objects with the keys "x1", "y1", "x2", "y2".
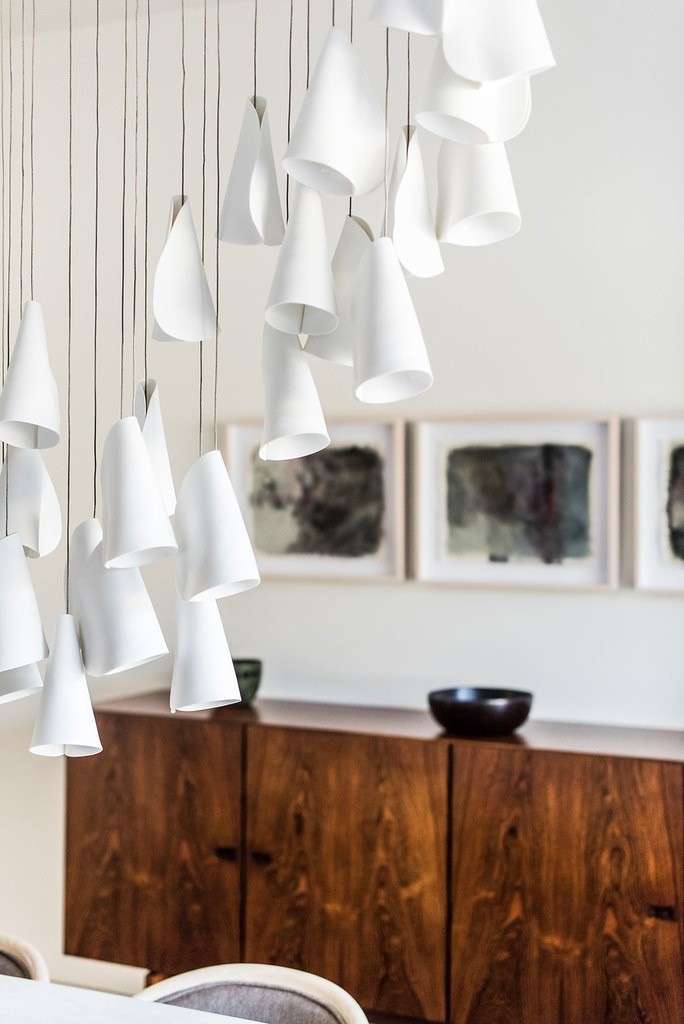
[{"x1": 231, "y1": 659, "x2": 262, "y2": 703}]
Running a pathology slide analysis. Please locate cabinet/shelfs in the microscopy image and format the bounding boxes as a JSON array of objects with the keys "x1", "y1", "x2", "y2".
[{"x1": 64, "y1": 687, "x2": 684, "y2": 1024}]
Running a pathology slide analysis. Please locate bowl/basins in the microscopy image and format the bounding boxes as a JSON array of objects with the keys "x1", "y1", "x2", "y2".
[{"x1": 428, "y1": 689, "x2": 532, "y2": 735}]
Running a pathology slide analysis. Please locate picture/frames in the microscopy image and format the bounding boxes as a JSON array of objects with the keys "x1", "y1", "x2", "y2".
[
  {"x1": 410, "y1": 413, "x2": 622, "y2": 593},
  {"x1": 629, "y1": 414, "x2": 684, "y2": 598},
  {"x1": 221, "y1": 416, "x2": 404, "y2": 585}
]
